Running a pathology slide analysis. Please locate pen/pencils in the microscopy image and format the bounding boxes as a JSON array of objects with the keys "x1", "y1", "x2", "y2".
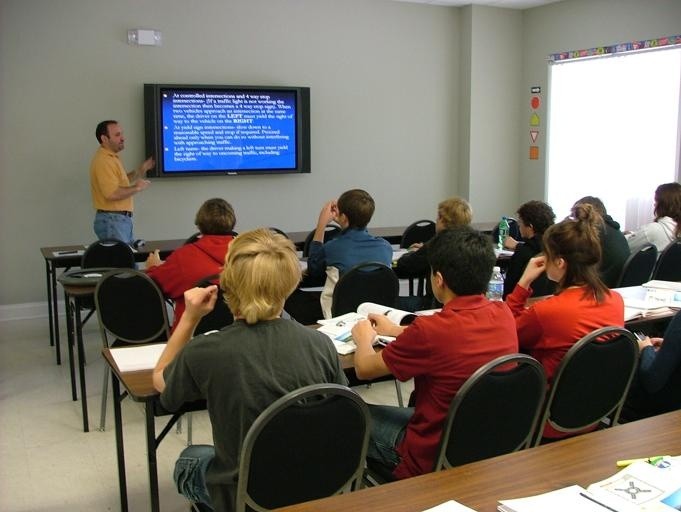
[
  {"x1": 59, "y1": 250, "x2": 78, "y2": 254},
  {"x1": 617, "y1": 455, "x2": 671, "y2": 467}
]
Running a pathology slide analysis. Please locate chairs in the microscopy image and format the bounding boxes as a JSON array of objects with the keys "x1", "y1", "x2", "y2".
[
  {"x1": 235, "y1": 380, "x2": 382, "y2": 512},
  {"x1": 93, "y1": 267, "x2": 184, "y2": 438},
  {"x1": 535, "y1": 326, "x2": 644, "y2": 453},
  {"x1": 77, "y1": 217, "x2": 681, "y2": 342},
  {"x1": 436, "y1": 351, "x2": 551, "y2": 474}
]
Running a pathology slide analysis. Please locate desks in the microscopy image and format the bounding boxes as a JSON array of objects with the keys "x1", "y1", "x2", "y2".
[
  {"x1": 102, "y1": 285, "x2": 681, "y2": 512},
  {"x1": 59, "y1": 242, "x2": 516, "y2": 432},
  {"x1": 276, "y1": 406, "x2": 681, "y2": 511},
  {"x1": 40, "y1": 221, "x2": 511, "y2": 363}
]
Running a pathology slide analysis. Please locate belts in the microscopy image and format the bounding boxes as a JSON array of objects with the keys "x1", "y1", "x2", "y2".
[{"x1": 98, "y1": 209, "x2": 133, "y2": 217}]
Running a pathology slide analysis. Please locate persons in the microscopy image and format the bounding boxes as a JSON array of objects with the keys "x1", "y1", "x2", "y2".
[
  {"x1": 637, "y1": 309, "x2": 681, "y2": 413},
  {"x1": 144, "y1": 198, "x2": 239, "y2": 332},
  {"x1": 502, "y1": 201, "x2": 558, "y2": 301},
  {"x1": 88, "y1": 120, "x2": 156, "y2": 245},
  {"x1": 351, "y1": 224, "x2": 519, "y2": 471},
  {"x1": 627, "y1": 182, "x2": 681, "y2": 264},
  {"x1": 396, "y1": 196, "x2": 473, "y2": 307},
  {"x1": 571, "y1": 196, "x2": 630, "y2": 288},
  {"x1": 505, "y1": 202, "x2": 624, "y2": 444},
  {"x1": 152, "y1": 228, "x2": 351, "y2": 512},
  {"x1": 308, "y1": 189, "x2": 393, "y2": 280}
]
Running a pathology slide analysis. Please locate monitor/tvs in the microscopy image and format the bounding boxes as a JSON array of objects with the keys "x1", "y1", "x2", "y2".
[{"x1": 144, "y1": 84, "x2": 311, "y2": 178}]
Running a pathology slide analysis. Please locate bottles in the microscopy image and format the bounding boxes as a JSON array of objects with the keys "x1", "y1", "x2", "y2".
[
  {"x1": 498, "y1": 216, "x2": 511, "y2": 246},
  {"x1": 488, "y1": 267, "x2": 506, "y2": 301}
]
